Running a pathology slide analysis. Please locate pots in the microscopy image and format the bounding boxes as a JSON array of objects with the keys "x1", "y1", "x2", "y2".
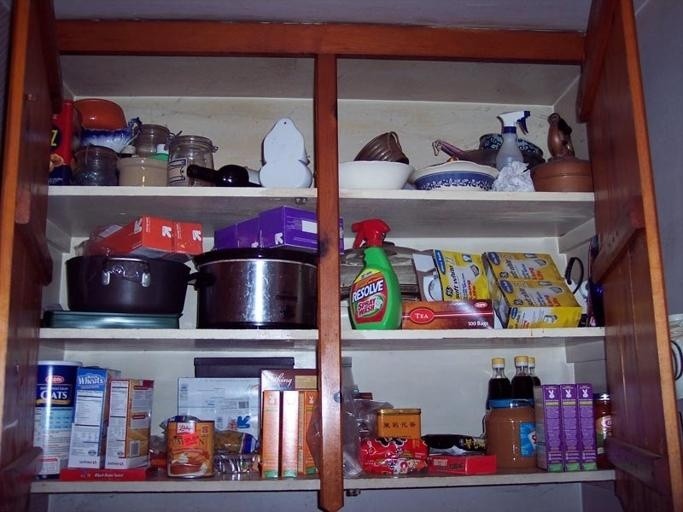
[
  {"x1": 64, "y1": 252, "x2": 192, "y2": 316},
  {"x1": 189, "y1": 248, "x2": 317, "y2": 330}
]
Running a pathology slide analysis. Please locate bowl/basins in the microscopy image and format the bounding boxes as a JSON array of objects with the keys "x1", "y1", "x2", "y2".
[
  {"x1": 459, "y1": 132, "x2": 545, "y2": 171},
  {"x1": 113, "y1": 157, "x2": 167, "y2": 186},
  {"x1": 81, "y1": 127, "x2": 134, "y2": 150},
  {"x1": 336, "y1": 160, "x2": 411, "y2": 192},
  {"x1": 69, "y1": 95, "x2": 127, "y2": 131},
  {"x1": 408, "y1": 160, "x2": 502, "y2": 192}
]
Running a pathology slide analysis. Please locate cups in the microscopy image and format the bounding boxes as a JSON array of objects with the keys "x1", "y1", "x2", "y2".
[
  {"x1": 352, "y1": 130, "x2": 411, "y2": 164},
  {"x1": 133, "y1": 124, "x2": 170, "y2": 157}
]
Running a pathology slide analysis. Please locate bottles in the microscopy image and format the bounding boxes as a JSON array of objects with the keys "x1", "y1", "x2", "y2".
[
  {"x1": 71, "y1": 143, "x2": 119, "y2": 186},
  {"x1": 183, "y1": 160, "x2": 259, "y2": 187},
  {"x1": 591, "y1": 390, "x2": 616, "y2": 470},
  {"x1": 340, "y1": 356, "x2": 354, "y2": 388},
  {"x1": 162, "y1": 134, "x2": 216, "y2": 188},
  {"x1": 484, "y1": 355, "x2": 542, "y2": 401}
]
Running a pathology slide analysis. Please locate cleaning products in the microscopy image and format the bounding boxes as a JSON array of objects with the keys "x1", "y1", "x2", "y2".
[
  {"x1": 495, "y1": 110, "x2": 531, "y2": 171},
  {"x1": 348, "y1": 219, "x2": 403, "y2": 329}
]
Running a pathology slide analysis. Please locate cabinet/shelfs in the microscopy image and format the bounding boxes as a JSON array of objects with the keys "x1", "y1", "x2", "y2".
[{"x1": 0, "y1": 0, "x2": 682, "y2": 512}]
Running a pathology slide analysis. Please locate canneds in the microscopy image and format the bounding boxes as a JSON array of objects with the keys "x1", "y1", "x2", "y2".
[
  {"x1": 166, "y1": 419, "x2": 214, "y2": 478},
  {"x1": 373, "y1": 409, "x2": 422, "y2": 442},
  {"x1": 31, "y1": 359, "x2": 82, "y2": 481},
  {"x1": 594, "y1": 391, "x2": 617, "y2": 470}
]
